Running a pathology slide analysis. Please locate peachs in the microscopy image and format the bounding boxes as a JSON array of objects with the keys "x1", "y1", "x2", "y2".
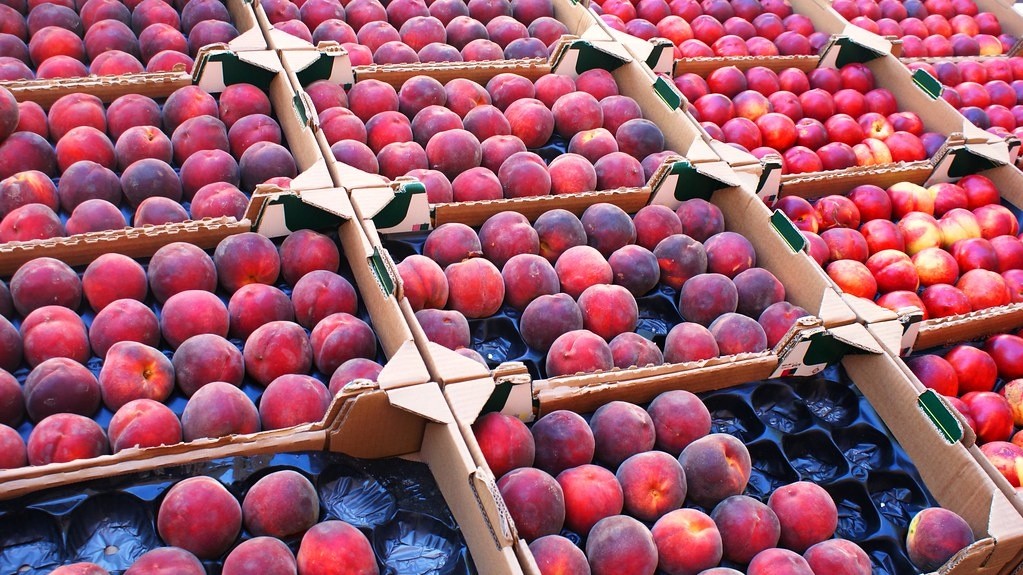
[
  {"x1": 0, "y1": 0, "x2": 977, "y2": 575},
  {"x1": 585, "y1": 0, "x2": 1023, "y2": 494}
]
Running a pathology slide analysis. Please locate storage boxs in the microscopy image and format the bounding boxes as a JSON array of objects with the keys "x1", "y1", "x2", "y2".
[{"x1": 0, "y1": 0, "x2": 1023, "y2": 575}]
